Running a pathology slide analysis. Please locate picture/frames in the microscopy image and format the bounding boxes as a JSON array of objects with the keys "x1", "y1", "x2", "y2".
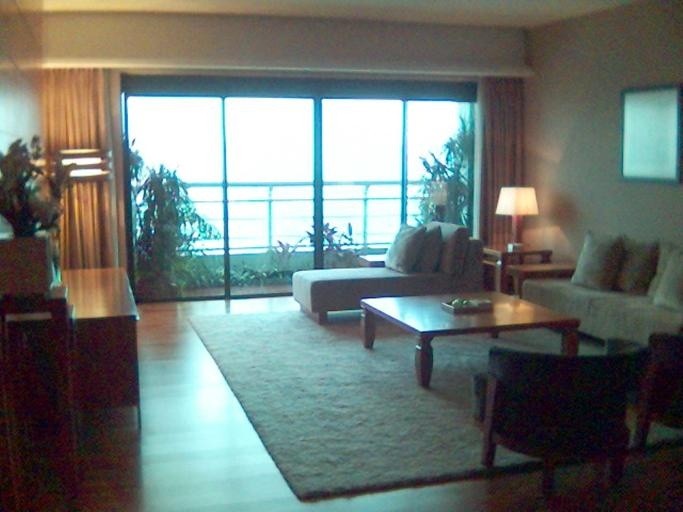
[{"x1": 618, "y1": 81, "x2": 682, "y2": 186}]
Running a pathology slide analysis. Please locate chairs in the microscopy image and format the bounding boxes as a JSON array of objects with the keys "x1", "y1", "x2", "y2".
[
  {"x1": 467, "y1": 332, "x2": 636, "y2": 503},
  {"x1": 289, "y1": 218, "x2": 485, "y2": 329},
  {"x1": 595, "y1": 314, "x2": 683, "y2": 474}
]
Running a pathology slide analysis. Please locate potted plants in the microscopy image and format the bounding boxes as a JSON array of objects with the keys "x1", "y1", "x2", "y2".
[
  {"x1": 35, "y1": 151, "x2": 75, "y2": 286},
  {"x1": 135, "y1": 164, "x2": 224, "y2": 299},
  {"x1": 0, "y1": 133, "x2": 53, "y2": 298}
]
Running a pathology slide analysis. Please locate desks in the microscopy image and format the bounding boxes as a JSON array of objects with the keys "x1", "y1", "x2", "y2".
[
  {"x1": 356, "y1": 250, "x2": 388, "y2": 266},
  {"x1": 480, "y1": 242, "x2": 554, "y2": 288},
  {"x1": 355, "y1": 290, "x2": 582, "y2": 389}
]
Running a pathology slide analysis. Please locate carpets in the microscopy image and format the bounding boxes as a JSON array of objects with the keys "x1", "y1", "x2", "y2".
[{"x1": 186, "y1": 306, "x2": 683, "y2": 501}]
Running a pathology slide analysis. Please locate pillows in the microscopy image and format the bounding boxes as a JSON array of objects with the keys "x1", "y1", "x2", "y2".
[
  {"x1": 415, "y1": 226, "x2": 443, "y2": 277},
  {"x1": 641, "y1": 235, "x2": 674, "y2": 294},
  {"x1": 568, "y1": 232, "x2": 624, "y2": 291},
  {"x1": 613, "y1": 242, "x2": 660, "y2": 294},
  {"x1": 384, "y1": 225, "x2": 424, "y2": 270},
  {"x1": 650, "y1": 251, "x2": 682, "y2": 309}
]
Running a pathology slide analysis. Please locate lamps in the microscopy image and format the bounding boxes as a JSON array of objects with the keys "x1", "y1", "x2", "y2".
[
  {"x1": 427, "y1": 179, "x2": 448, "y2": 222},
  {"x1": 56, "y1": 145, "x2": 110, "y2": 183},
  {"x1": 493, "y1": 186, "x2": 539, "y2": 251}
]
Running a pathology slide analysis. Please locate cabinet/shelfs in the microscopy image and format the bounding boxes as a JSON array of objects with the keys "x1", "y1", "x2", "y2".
[
  {"x1": 5, "y1": 266, "x2": 142, "y2": 433},
  {"x1": 0, "y1": 285, "x2": 84, "y2": 511}
]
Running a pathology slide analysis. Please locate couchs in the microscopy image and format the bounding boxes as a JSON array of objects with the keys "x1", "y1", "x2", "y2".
[{"x1": 506, "y1": 232, "x2": 683, "y2": 348}]
